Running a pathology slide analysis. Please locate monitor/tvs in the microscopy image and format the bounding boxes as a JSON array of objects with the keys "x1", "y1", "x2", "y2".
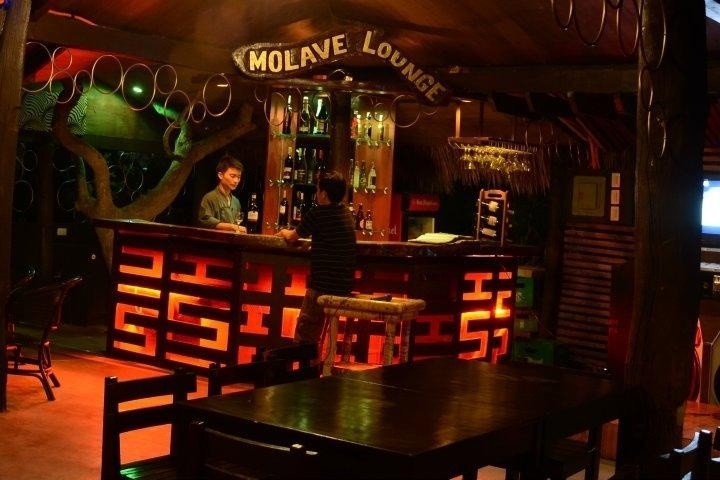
[{"x1": 697, "y1": 176, "x2": 720, "y2": 245}]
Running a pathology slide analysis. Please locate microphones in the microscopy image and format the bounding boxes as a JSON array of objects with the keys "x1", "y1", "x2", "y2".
[{"x1": 373, "y1": 293, "x2": 393, "y2": 300}]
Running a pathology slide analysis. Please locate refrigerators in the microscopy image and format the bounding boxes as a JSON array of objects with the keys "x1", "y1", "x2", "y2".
[{"x1": 388, "y1": 192, "x2": 440, "y2": 241}]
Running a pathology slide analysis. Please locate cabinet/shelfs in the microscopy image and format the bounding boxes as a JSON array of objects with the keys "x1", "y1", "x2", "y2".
[{"x1": 272, "y1": 129, "x2": 389, "y2": 236}]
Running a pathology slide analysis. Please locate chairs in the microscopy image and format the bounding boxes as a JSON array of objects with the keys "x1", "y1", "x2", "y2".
[
  {"x1": 186, "y1": 413, "x2": 325, "y2": 478},
  {"x1": 2, "y1": 270, "x2": 82, "y2": 412},
  {"x1": 503, "y1": 403, "x2": 604, "y2": 479},
  {"x1": 257, "y1": 340, "x2": 326, "y2": 384},
  {"x1": 656, "y1": 430, "x2": 713, "y2": 479},
  {"x1": 102, "y1": 368, "x2": 196, "y2": 479},
  {"x1": 7, "y1": 261, "x2": 34, "y2": 298},
  {"x1": 209, "y1": 361, "x2": 281, "y2": 395}
]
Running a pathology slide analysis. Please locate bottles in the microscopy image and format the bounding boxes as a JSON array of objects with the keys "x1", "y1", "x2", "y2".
[
  {"x1": 282, "y1": 95, "x2": 384, "y2": 142},
  {"x1": 278, "y1": 191, "x2": 373, "y2": 233},
  {"x1": 246, "y1": 192, "x2": 261, "y2": 231},
  {"x1": 475, "y1": 212, "x2": 514, "y2": 233},
  {"x1": 473, "y1": 224, "x2": 514, "y2": 244},
  {"x1": 477, "y1": 197, "x2": 515, "y2": 220},
  {"x1": 282, "y1": 146, "x2": 377, "y2": 194}
]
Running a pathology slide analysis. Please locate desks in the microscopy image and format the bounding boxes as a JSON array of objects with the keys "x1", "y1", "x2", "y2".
[{"x1": 168, "y1": 352, "x2": 624, "y2": 477}]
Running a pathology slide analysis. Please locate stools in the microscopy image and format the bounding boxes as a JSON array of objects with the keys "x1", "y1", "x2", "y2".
[{"x1": 318, "y1": 293, "x2": 424, "y2": 372}]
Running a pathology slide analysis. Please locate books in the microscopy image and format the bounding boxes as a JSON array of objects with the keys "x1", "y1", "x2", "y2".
[{"x1": 407, "y1": 231, "x2": 476, "y2": 246}]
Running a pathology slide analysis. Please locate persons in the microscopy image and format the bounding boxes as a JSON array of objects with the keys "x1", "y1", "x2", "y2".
[
  {"x1": 196, "y1": 153, "x2": 253, "y2": 237},
  {"x1": 274, "y1": 166, "x2": 359, "y2": 351}
]
